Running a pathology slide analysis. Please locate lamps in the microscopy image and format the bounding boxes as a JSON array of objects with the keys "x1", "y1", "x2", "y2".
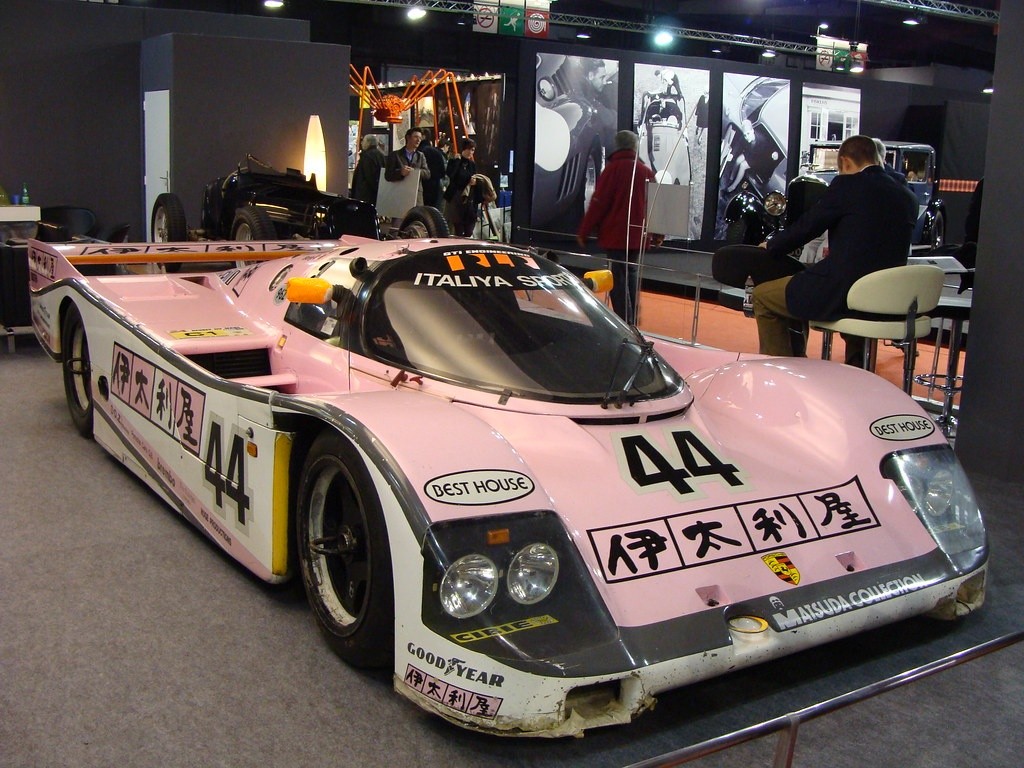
[
  {"x1": 903, "y1": 10, "x2": 922, "y2": 25},
  {"x1": 849, "y1": 62, "x2": 865, "y2": 73},
  {"x1": 762, "y1": 49, "x2": 777, "y2": 58},
  {"x1": 980, "y1": 80, "x2": 994, "y2": 94},
  {"x1": 576, "y1": 26, "x2": 592, "y2": 38},
  {"x1": 836, "y1": 60, "x2": 845, "y2": 70}
]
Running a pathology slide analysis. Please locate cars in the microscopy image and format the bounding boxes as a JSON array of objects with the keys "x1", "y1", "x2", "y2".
[
  {"x1": 725, "y1": 135, "x2": 949, "y2": 253},
  {"x1": 151, "y1": 156, "x2": 450, "y2": 271}
]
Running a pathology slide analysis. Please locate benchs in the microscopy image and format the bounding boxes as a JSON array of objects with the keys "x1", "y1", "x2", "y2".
[{"x1": 914, "y1": 269, "x2": 976, "y2": 438}]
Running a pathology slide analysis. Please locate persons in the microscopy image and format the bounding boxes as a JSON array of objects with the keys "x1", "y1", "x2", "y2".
[
  {"x1": 575, "y1": 129, "x2": 664, "y2": 329},
  {"x1": 351, "y1": 128, "x2": 483, "y2": 238},
  {"x1": 754, "y1": 136, "x2": 982, "y2": 358},
  {"x1": 571, "y1": 58, "x2": 774, "y2": 194}
]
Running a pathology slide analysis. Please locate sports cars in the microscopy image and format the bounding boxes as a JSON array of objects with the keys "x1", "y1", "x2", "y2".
[{"x1": 21, "y1": 221, "x2": 992, "y2": 739}]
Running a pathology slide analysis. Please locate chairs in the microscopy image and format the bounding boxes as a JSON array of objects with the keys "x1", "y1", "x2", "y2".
[{"x1": 809, "y1": 265, "x2": 945, "y2": 398}]
